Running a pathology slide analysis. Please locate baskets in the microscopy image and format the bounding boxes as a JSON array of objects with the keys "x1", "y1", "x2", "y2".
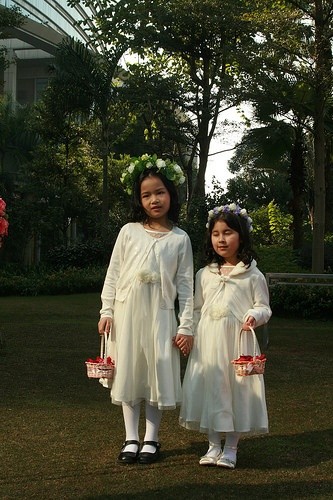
[
  {"x1": 85, "y1": 332, "x2": 115, "y2": 378},
  {"x1": 230, "y1": 326, "x2": 267, "y2": 376}
]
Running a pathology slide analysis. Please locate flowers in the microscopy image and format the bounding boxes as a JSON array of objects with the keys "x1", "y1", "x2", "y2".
[
  {"x1": 137, "y1": 268, "x2": 159, "y2": 284},
  {"x1": 207, "y1": 303, "x2": 230, "y2": 320},
  {"x1": 119, "y1": 153, "x2": 186, "y2": 197},
  {"x1": 205, "y1": 203, "x2": 254, "y2": 234}
]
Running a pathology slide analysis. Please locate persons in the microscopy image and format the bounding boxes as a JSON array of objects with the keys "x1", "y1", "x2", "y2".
[
  {"x1": 97, "y1": 154, "x2": 195, "y2": 466},
  {"x1": 171, "y1": 203, "x2": 274, "y2": 469}
]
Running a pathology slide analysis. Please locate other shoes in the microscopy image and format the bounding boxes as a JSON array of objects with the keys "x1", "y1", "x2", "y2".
[
  {"x1": 199, "y1": 442, "x2": 223, "y2": 465},
  {"x1": 217, "y1": 446, "x2": 238, "y2": 468}
]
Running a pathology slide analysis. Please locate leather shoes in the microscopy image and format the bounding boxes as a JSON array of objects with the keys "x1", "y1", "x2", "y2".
[
  {"x1": 116, "y1": 440, "x2": 141, "y2": 463},
  {"x1": 138, "y1": 441, "x2": 162, "y2": 463}
]
